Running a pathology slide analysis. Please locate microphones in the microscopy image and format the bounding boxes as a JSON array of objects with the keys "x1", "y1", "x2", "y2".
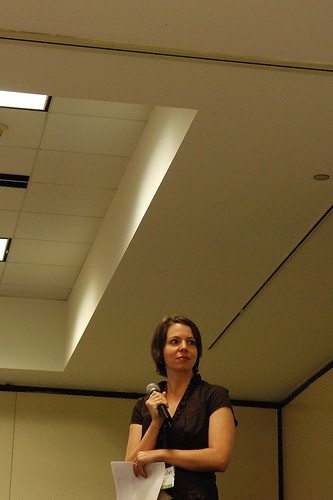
[{"x1": 146, "y1": 382, "x2": 172, "y2": 425}]
